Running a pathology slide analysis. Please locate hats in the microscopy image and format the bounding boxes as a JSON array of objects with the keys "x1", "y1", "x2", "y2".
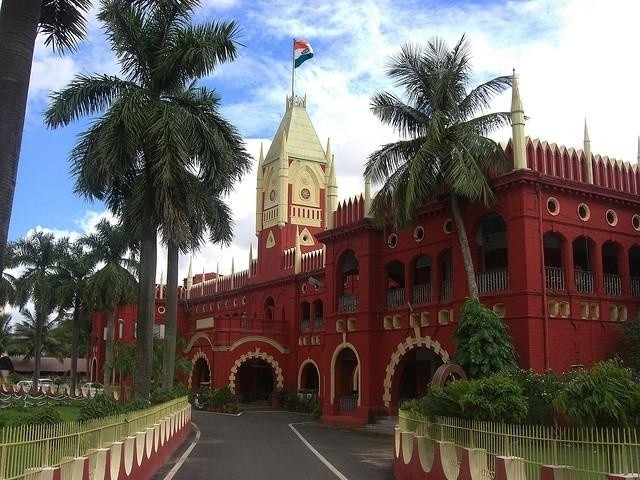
[{"x1": 17, "y1": 378, "x2": 106, "y2": 394}]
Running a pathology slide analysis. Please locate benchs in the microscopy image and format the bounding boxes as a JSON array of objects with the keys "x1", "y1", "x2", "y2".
[{"x1": 294, "y1": 39, "x2": 314, "y2": 69}]
